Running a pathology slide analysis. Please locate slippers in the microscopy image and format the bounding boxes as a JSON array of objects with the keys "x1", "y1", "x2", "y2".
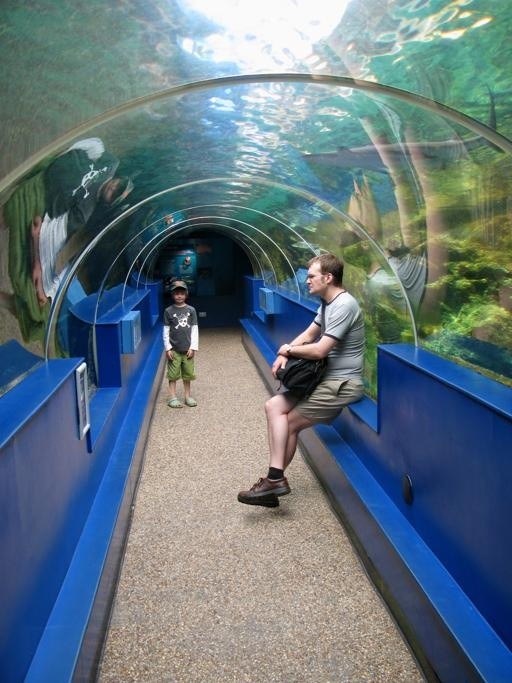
[
  {"x1": 186, "y1": 396, "x2": 197, "y2": 406},
  {"x1": 168, "y1": 398, "x2": 183, "y2": 407}
]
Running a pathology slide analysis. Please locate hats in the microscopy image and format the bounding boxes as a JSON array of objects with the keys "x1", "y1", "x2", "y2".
[
  {"x1": 170, "y1": 280, "x2": 188, "y2": 290},
  {"x1": 110, "y1": 175, "x2": 135, "y2": 208}
]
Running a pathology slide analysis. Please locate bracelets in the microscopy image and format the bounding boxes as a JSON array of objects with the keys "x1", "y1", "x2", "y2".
[{"x1": 285, "y1": 343, "x2": 295, "y2": 358}]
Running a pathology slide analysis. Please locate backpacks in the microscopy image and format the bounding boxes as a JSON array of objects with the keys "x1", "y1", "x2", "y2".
[{"x1": 446, "y1": 246, "x2": 500, "y2": 310}]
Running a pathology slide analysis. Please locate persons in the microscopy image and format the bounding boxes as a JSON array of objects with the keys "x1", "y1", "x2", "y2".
[
  {"x1": 304, "y1": 32, "x2": 452, "y2": 345},
  {"x1": 237, "y1": 252, "x2": 367, "y2": 508},
  {"x1": 161, "y1": 280, "x2": 200, "y2": 409},
  {"x1": 28, "y1": 133, "x2": 136, "y2": 307}
]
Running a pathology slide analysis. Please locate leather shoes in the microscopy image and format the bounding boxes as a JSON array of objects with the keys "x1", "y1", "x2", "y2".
[
  {"x1": 238, "y1": 496, "x2": 279, "y2": 507},
  {"x1": 239, "y1": 477, "x2": 291, "y2": 499}
]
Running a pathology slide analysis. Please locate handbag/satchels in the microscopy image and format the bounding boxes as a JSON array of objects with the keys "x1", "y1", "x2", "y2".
[{"x1": 276, "y1": 341, "x2": 330, "y2": 401}]
